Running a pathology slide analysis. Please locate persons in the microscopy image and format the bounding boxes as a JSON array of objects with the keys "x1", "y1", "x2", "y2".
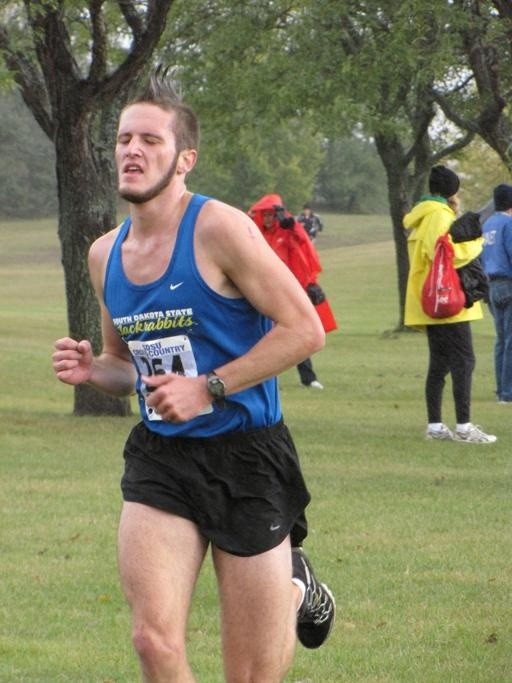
[
  {"x1": 400, "y1": 163, "x2": 498, "y2": 444},
  {"x1": 295, "y1": 202, "x2": 323, "y2": 244},
  {"x1": 477, "y1": 183, "x2": 512, "y2": 402},
  {"x1": 245, "y1": 193, "x2": 337, "y2": 390},
  {"x1": 49, "y1": 93, "x2": 329, "y2": 681}
]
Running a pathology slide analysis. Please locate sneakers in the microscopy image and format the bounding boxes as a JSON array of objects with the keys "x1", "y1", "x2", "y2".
[
  {"x1": 454, "y1": 422, "x2": 497, "y2": 444},
  {"x1": 291, "y1": 545, "x2": 336, "y2": 649},
  {"x1": 426, "y1": 422, "x2": 457, "y2": 440},
  {"x1": 310, "y1": 380, "x2": 323, "y2": 388}
]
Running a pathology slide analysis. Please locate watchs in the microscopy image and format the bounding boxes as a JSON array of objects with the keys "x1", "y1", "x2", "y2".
[{"x1": 206, "y1": 370, "x2": 226, "y2": 401}]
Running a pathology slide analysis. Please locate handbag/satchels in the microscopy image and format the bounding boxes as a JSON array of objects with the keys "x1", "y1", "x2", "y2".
[{"x1": 422, "y1": 232, "x2": 466, "y2": 318}]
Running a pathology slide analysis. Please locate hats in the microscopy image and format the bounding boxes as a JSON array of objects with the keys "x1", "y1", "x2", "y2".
[
  {"x1": 430, "y1": 165, "x2": 459, "y2": 197},
  {"x1": 493, "y1": 183, "x2": 512, "y2": 211}
]
